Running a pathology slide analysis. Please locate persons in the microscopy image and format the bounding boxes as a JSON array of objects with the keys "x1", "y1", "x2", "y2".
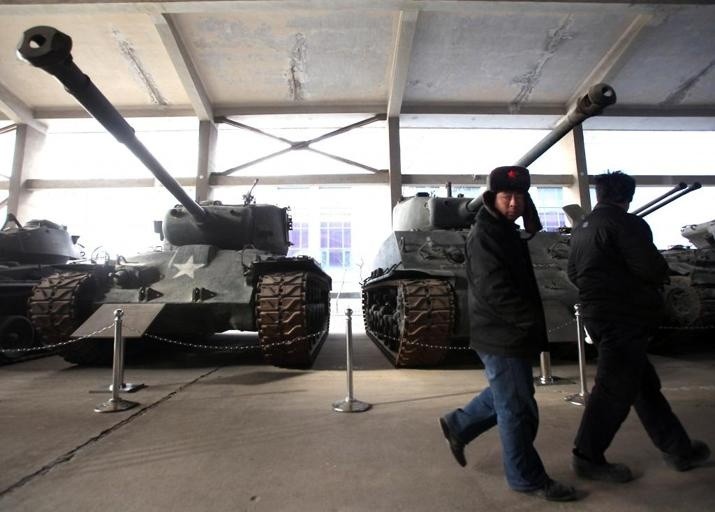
[
  {"x1": 566, "y1": 171, "x2": 711, "y2": 483},
  {"x1": 439, "y1": 166, "x2": 577, "y2": 501}
]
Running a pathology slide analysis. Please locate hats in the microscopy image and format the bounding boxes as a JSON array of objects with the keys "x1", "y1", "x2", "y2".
[{"x1": 482, "y1": 166, "x2": 542, "y2": 234}]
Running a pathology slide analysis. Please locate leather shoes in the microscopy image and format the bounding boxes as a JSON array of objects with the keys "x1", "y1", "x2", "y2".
[
  {"x1": 519, "y1": 473, "x2": 577, "y2": 503},
  {"x1": 570, "y1": 447, "x2": 633, "y2": 484},
  {"x1": 663, "y1": 440, "x2": 711, "y2": 472},
  {"x1": 439, "y1": 416, "x2": 468, "y2": 467}
]
